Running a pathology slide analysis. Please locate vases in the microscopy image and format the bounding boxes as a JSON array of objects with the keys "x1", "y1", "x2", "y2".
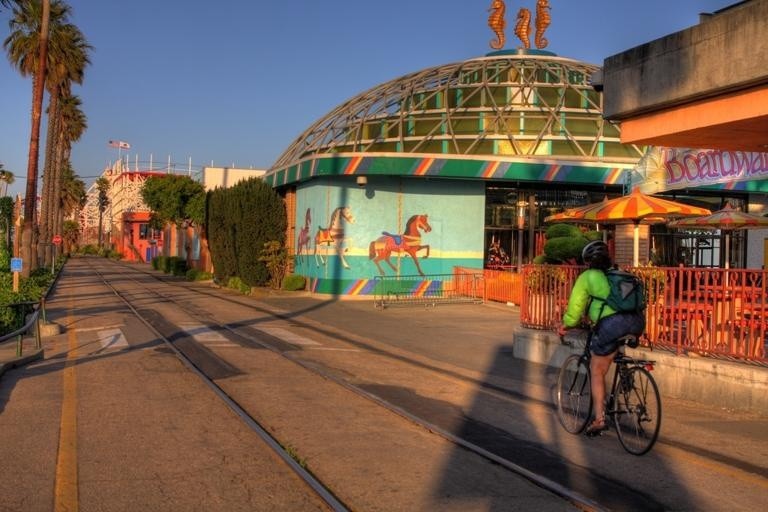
[
  {"x1": 638, "y1": 302, "x2": 659, "y2": 348},
  {"x1": 525, "y1": 292, "x2": 556, "y2": 330}
]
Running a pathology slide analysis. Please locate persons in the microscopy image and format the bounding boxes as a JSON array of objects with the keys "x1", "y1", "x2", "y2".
[{"x1": 555, "y1": 240, "x2": 647, "y2": 439}]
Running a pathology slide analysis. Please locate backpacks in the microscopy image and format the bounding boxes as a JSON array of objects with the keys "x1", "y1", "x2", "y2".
[{"x1": 600, "y1": 267, "x2": 645, "y2": 315}]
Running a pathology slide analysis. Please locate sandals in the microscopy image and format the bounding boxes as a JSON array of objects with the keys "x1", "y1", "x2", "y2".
[{"x1": 584, "y1": 419, "x2": 608, "y2": 437}]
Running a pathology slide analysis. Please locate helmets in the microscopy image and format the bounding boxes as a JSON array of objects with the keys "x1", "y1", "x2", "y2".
[{"x1": 580, "y1": 240, "x2": 609, "y2": 262}]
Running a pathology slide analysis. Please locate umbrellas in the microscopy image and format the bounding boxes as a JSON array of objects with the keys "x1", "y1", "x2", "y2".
[
  {"x1": 564, "y1": 187, "x2": 713, "y2": 268},
  {"x1": 664, "y1": 201, "x2": 767, "y2": 294},
  {"x1": 542, "y1": 195, "x2": 675, "y2": 244}
]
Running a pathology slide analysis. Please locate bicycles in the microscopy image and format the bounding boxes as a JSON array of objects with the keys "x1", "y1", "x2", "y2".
[{"x1": 553, "y1": 320, "x2": 663, "y2": 459}]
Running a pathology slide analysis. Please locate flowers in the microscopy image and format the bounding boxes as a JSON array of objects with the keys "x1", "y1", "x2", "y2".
[
  {"x1": 620, "y1": 261, "x2": 665, "y2": 304},
  {"x1": 519, "y1": 264, "x2": 567, "y2": 294}
]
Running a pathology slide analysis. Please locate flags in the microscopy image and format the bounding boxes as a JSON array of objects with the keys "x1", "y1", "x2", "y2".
[
  {"x1": 119, "y1": 140, "x2": 130, "y2": 151},
  {"x1": 107, "y1": 139, "x2": 120, "y2": 149}
]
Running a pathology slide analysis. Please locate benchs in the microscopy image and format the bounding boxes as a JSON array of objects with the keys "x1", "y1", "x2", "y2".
[{"x1": 658, "y1": 285, "x2": 768, "y2": 363}]
